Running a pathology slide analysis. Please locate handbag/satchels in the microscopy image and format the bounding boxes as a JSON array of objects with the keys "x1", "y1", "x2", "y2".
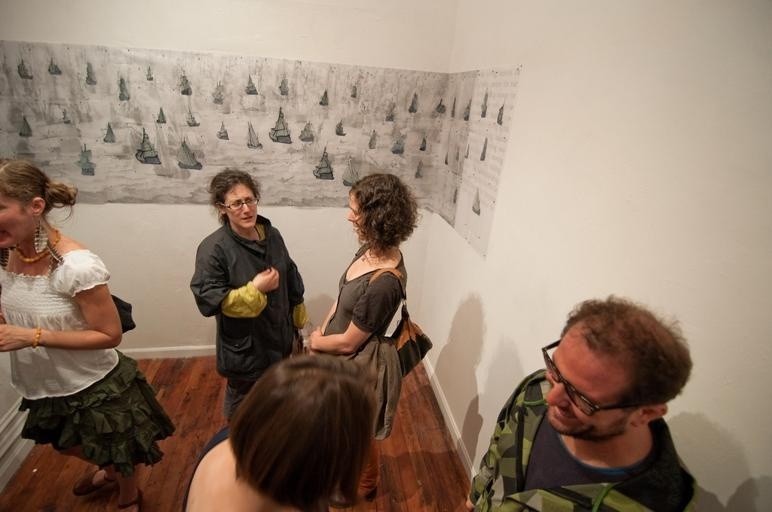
[
  {"x1": 111, "y1": 295, "x2": 138, "y2": 334},
  {"x1": 368, "y1": 267, "x2": 434, "y2": 379}
]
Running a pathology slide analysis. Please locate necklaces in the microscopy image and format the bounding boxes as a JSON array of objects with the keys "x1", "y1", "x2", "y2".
[
  {"x1": 14, "y1": 227, "x2": 61, "y2": 263},
  {"x1": 361, "y1": 252, "x2": 374, "y2": 262}
]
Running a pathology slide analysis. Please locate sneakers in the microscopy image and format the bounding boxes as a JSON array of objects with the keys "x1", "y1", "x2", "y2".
[{"x1": 329, "y1": 484, "x2": 378, "y2": 510}]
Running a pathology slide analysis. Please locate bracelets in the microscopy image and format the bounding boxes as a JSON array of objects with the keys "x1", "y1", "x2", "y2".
[{"x1": 32, "y1": 326, "x2": 42, "y2": 349}]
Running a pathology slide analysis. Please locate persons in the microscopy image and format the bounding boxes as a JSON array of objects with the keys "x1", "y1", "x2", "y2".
[
  {"x1": 191, "y1": 170, "x2": 307, "y2": 423},
  {"x1": 308, "y1": 174, "x2": 417, "y2": 508},
  {"x1": 465, "y1": 300, "x2": 695, "y2": 512},
  {"x1": 0, "y1": 156, "x2": 176, "y2": 512},
  {"x1": 183, "y1": 354, "x2": 377, "y2": 511}
]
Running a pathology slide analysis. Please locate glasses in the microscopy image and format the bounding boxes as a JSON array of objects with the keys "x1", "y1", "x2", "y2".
[
  {"x1": 218, "y1": 197, "x2": 260, "y2": 210},
  {"x1": 541, "y1": 337, "x2": 639, "y2": 417}
]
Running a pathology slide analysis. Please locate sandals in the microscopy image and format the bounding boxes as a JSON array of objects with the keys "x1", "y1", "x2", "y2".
[
  {"x1": 112, "y1": 487, "x2": 143, "y2": 512},
  {"x1": 73, "y1": 465, "x2": 115, "y2": 496}
]
